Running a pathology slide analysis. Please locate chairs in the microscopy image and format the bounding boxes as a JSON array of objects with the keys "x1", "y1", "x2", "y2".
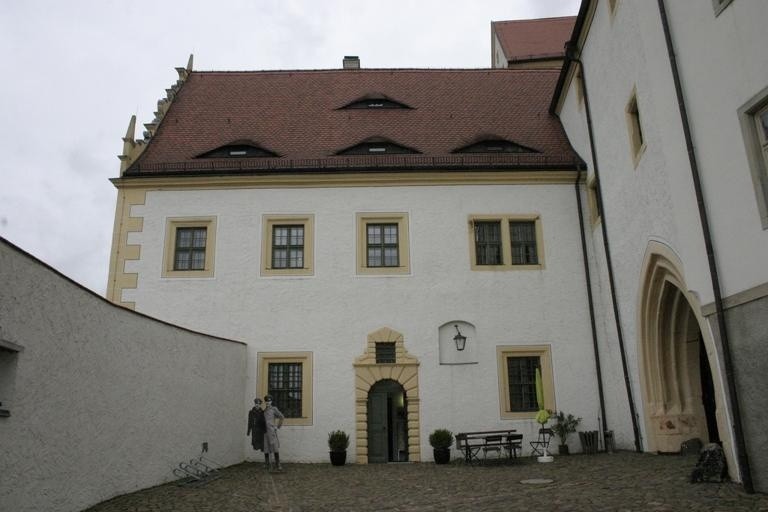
[
  {"x1": 530, "y1": 428, "x2": 558, "y2": 460},
  {"x1": 454, "y1": 434, "x2": 523, "y2": 465}
]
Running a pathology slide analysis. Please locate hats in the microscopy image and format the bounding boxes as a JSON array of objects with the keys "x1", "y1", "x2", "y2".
[
  {"x1": 253, "y1": 398, "x2": 263, "y2": 404},
  {"x1": 263, "y1": 395, "x2": 273, "y2": 401}
]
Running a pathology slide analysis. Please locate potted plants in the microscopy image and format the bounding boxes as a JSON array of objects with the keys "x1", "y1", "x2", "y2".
[
  {"x1": 550, "y1": 409, "x2": 584, "y2": 455},
  {"x1": 327, "y1": 429, "x2": 351, "y2": 466},
  {"x1": 429, "y1": 427, "x2": 455, "y2": 464}
]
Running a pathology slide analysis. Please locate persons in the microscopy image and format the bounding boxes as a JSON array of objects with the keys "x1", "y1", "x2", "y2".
[
  {"x1": 261, "y1": 395, "x2": 284, "y2": 471},
  {"x1": 246, "y1": 397, "x2": 267, "y2": 451}
]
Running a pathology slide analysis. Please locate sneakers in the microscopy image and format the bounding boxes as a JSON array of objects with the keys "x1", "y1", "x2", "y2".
[{"x1": 275, "y1": 460, "x2": 282, "y2": 470}]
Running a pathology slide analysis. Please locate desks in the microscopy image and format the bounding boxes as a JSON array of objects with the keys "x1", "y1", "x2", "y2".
[{"x1": 458, "y1": 429, "x2": 517, "y2": 466}]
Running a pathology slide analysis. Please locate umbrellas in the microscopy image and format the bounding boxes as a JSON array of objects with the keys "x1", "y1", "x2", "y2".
[{"x1": 534, "y1": 365, "x2": 551, "y2": 457}]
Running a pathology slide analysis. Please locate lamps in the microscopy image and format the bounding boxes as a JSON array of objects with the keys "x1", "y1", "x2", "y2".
[{"x1": 452, "y1": 324, "x2": 466, "y2": 351}]
